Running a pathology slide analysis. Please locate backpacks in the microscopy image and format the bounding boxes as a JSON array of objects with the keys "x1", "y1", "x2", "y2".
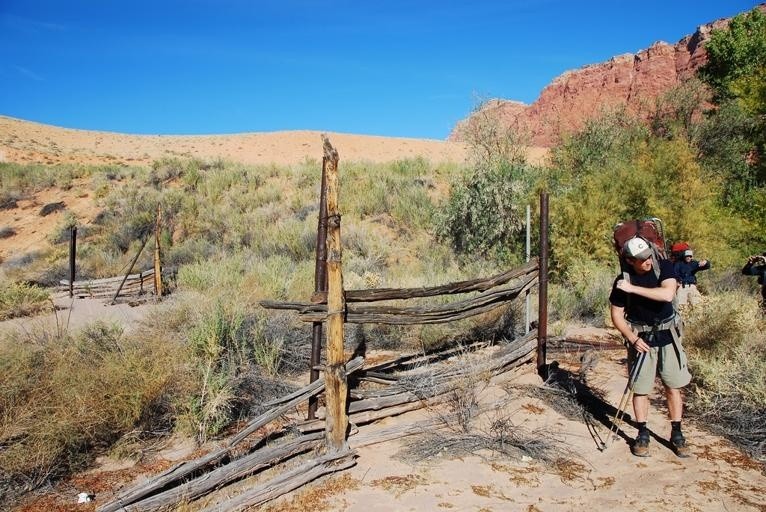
[
  {"x1": 672, "y1": 242, "x2": 688, "y2": 260},
  {"x1": 614, "y1": 217, "x2": 667, "y2": 282}
]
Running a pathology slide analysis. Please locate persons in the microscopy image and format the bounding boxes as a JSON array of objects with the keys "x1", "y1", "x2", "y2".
[
  {"x1": 609, "y1": 236, "x2": 692, "y2": 458},
  {"x1": 674, "y1": 249, "x2": 710, "y2": 305},
  {"x1": 741, "y1": 251, "x2": 766, "y2": 307}
]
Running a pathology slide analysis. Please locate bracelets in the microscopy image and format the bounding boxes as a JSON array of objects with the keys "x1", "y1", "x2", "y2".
[{"x1": 634, "y1": 336, "x2": 641, "y2": 344}]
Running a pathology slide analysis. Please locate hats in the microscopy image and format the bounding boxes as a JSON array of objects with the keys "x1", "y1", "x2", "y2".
[
  {"x1": 623, "y1": 238, "x2": 653, "y2": 259},
  {"x1": 684, "y1": 250, "x2": 693, "y2": 257}
]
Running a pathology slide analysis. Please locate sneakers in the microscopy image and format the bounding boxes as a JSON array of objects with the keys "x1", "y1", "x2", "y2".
[
  {"x1": 670, "y1": 437, "x2": 690, "y2": 457},
  {"x1": 633, "y1": 433, "x2": 649, "y2": 455}
]
